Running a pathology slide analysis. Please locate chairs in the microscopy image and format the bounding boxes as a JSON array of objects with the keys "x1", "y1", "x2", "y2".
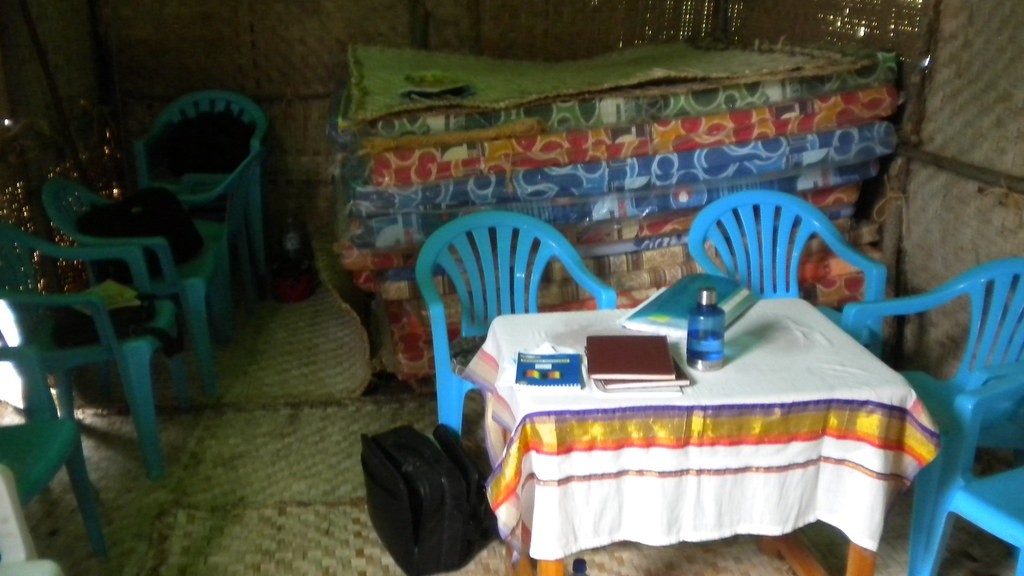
[
  {"x1": 413, "y1": 187, "x2": 1024, "y2": 576},
  {"x1": 0, "y1": 90, "x2": 273, "y2": 561}
]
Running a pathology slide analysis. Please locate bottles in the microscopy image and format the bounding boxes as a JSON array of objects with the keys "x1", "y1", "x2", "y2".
[{"x1": 686, "y1": 287, "x2": 726, "y2": 370}]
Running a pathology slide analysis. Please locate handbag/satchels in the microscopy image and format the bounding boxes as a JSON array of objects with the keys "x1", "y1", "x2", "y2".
[
  {"x1": 79, "y1": 187, "x2": 203, "y2": 284},
  {"x1": 361, "y1": 426, "x2": 467, "y2": 576}
]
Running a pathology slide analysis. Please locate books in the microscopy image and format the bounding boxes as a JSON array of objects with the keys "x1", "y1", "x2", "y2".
[
  {"x1": 585, "y1": 336, "x2": 690, "y2": 395},
  {"x1": 514, "y1": 354, "x2": 583, "y2": 392}
]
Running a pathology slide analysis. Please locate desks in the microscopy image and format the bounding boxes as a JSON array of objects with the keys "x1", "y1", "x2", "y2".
[{"x1": 459, "y1": 299, "x2": 942, "y2": 576}]
